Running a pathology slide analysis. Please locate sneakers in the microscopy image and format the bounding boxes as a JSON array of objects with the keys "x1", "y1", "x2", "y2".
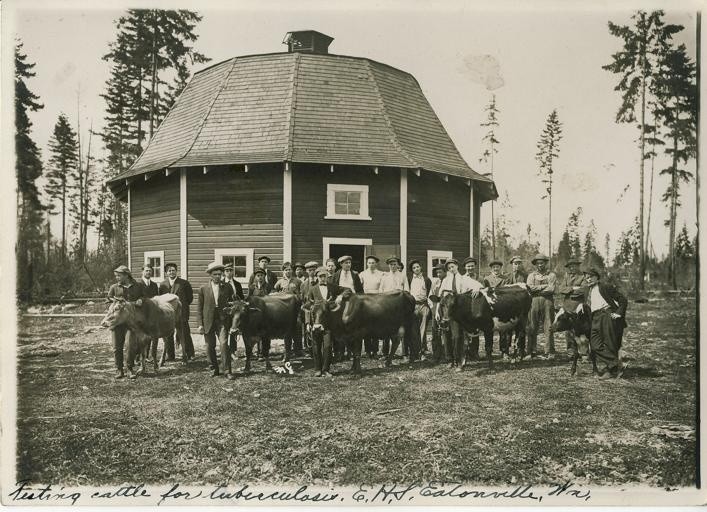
[
  {"x1": 126, "y1": 371, "x2": 137, "y2": 379},
  {"x1": 315, "y1": 370, "x2": 332, "y2": 377},
  {"x1": 115, "y1": 372, "x2": 124, "y2": 379},
  {"x1": 292, "y1": 345, "x2": 433, "y2": 359},
  {"x1": 230, "y1": 352, "x2": 239, "y2": 361},
  {"x1": 598, "y1": 372, "x2": 612, "y2": 381},
  {"x1": 225, "y1": 373, "x2": 235, "y2": 380},
  {"x1": 502, "y1": 352, "x2": 554, "y2": 363},
  {"x1": 209, "y1": 372, "x2": 219, "y2": 378}
]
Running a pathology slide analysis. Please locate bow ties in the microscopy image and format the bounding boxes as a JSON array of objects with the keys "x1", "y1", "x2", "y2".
[{"x1": 320, "y1": 284, "x2": 327, "y2": 287}]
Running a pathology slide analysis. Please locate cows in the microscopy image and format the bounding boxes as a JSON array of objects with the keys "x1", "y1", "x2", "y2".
[
  {"x1": 429, "y1": 283, "x2": 533, "y2": 372},
  {"x1": 101, "y1": 293, "x2": 188, "y2": 375},
  {"x1": 301, "y1": 290, "x2": 421, "y2": 380},
  {"x1": 223, "y1": 291, "x2": 302, "y2": 374},
  {"x1": 549, "y1": 303, "x2": 600, "y2": 379}
]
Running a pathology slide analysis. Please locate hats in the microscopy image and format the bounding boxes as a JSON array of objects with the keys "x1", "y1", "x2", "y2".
[
  {"x1": 114, "y1": 265, "x2": 133, "y2": 279},
  {"x1": 223, "y1": 263, "x2": 233, "y2": 270},
  {"x1": 365, "y1": 256, "x2": 581, "y2": 273},
  {"x1": 583, "y1": 267, "x2": 600, "y2": 281},
  {"x1": 253, "y1": 254, "x2": 353, "y2": 278},
  {"x1": 205, "y1": 262, "x2": 225, "y2": 273}
]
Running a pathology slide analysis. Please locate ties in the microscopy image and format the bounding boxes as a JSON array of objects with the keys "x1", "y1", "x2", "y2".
[
  {"x1": 452, "y1": 273, "x2": 457, "y2": 296},
  {"x1": 586, "y1": 287, "x2": 592, "y2": 307}
]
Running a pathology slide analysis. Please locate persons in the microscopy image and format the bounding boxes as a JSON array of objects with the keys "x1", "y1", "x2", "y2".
[{"x1": 105, "y1": 253, "x2": 629, "y2": 382}]
